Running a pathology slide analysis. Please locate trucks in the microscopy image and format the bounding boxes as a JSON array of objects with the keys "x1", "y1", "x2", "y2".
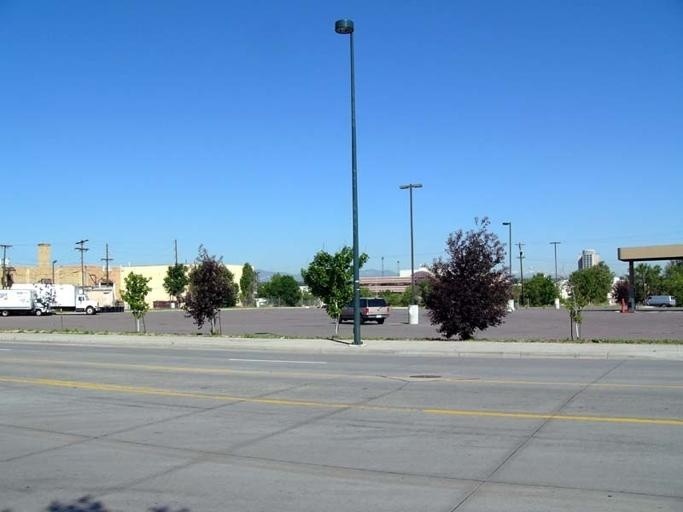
[{"x1": 0, "y1": 284, "x2": 99, "y2": 317}]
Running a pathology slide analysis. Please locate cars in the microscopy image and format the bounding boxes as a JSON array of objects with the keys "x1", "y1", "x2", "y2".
[{"x1": 338, "y1": 297, "x2": 389, "y2": 325}]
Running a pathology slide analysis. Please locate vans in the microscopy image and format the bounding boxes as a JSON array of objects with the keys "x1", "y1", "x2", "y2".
[{"x1": 643, "y1": 295, "x2": 675, "y2": 307}]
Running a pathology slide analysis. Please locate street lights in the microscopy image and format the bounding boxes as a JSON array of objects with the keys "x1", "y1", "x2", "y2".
[
  {"x1": 501, "y1": 223, "x2": 514, "y2": 312},
  {"x1": 51, "y1": 259, "x2": 58, "y2": 284},
  {"x1": 399, "y1": 184, "x2": 422, "y2": 327},
  {"x1": 549, "y1": 242, "x2": 561, "y2": 310},
  {"x1": 334, "y1": 18, "x2": 361, "y2": 345}
]
